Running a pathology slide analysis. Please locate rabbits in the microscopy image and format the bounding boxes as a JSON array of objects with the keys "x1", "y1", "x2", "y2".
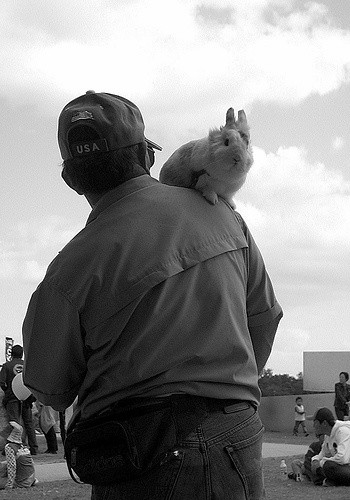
[{"x1": 160, "y1": 107, "x2": 255, "y2": 211}]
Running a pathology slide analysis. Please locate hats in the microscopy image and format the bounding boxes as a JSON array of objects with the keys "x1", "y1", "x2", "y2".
[
  {"x1": 12, "y1": 345, "x2": 23, "y2": 357},
  {"x1": 305, "y1": 406, "x2": 336, "y2": 422},
  {"x1": 58, "y1": 90, "x2": 162, "y2": 161},
  {"x1": 0, "y1": 421, "x2": 23, "y2": 443}
]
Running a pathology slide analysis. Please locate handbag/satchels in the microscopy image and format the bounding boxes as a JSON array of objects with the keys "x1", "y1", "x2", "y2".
[{"x1": 64, "y1": 398, "x2": 226, "y2": 484}]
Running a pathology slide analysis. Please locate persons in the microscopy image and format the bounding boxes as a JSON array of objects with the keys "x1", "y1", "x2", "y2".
[
  {"x1": 36, "y1": 400, "x2": 58, "y2": 453},
  {"x1": 303, "y1": 407, "x2": 350, "y2": 487},
  {"x1": 0, "y1": 345, "x2": 39, "y2": 454},
  {"x1": 292, "y1": 396, "x2": 309, "y2": 437},
  {"x1": 0, "y1": 421, "x2": 37, "y2": 488},
  {"x1": 23, "y1": 89, "x2": 282, "y2": 500},
  {"x1": 333, "y1": 372, "x2": 350, "y2": 421}
]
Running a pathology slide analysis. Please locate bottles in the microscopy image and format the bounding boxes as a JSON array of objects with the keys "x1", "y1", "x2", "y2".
[{"x1": 280, "y1": 460, "x2": 287, "y2": 481}]
